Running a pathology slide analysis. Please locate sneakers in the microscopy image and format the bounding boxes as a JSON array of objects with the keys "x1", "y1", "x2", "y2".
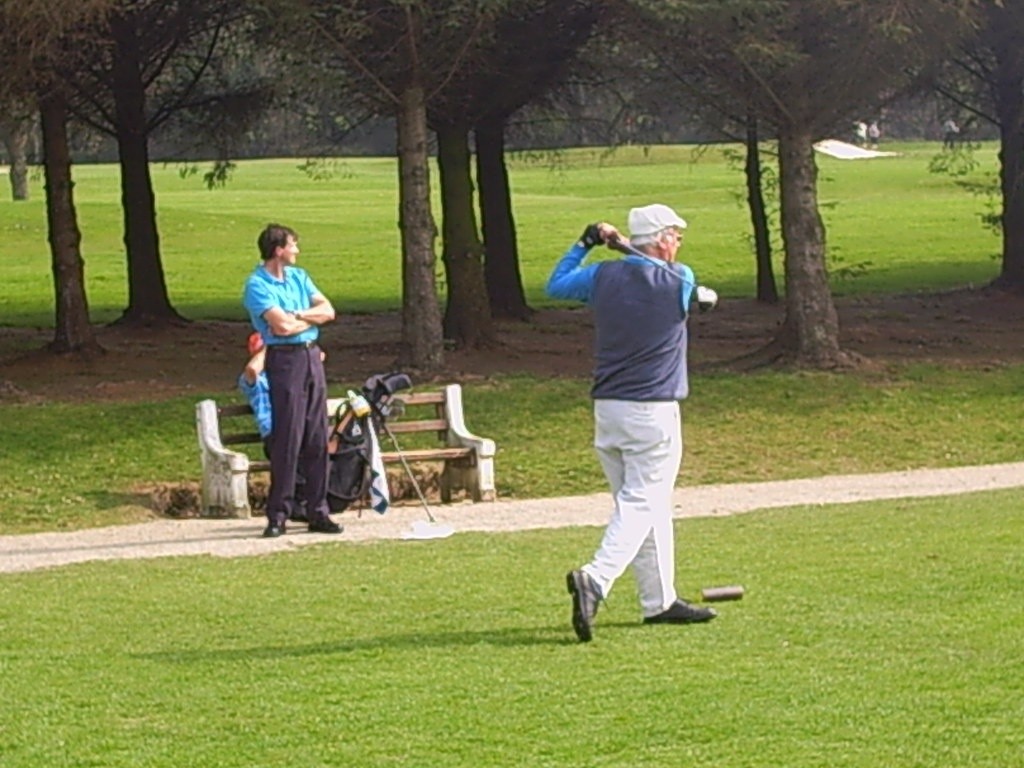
[
  {"x1": 308, "y1": 517, "x2": 342, "y2": 532},
  {"x1": 644, "y1": 597, "x2": 717, "y2": 625},
  {"x1": 266, "y1": 521, "x2": 286, "y2": 535},
  {"x1": 567, "y1": 569, "x2": 602, "y2": 641}
]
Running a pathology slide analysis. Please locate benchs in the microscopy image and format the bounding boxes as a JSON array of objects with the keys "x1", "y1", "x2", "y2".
[{"x1": 195, "y1": 383, "x2": 497, "y2": 520}]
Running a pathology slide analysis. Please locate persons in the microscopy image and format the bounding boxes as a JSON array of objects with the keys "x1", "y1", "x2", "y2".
[
  {"x1": 853, "y1": 117, "x2": 881, "y2": 149},
  {"x1": 238, "y1": 223, "x2": 343, "y2": 537},
  {"x1": 943, "y1": 114, "x2": 959, "y2": 149},
  {"x1": 544, "y1": 204, "x2": 717, "y2": 642}
]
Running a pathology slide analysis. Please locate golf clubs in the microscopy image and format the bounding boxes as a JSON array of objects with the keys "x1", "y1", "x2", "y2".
[{"x1": 605, "y1": 233, "x2": 719, "y2": 316}]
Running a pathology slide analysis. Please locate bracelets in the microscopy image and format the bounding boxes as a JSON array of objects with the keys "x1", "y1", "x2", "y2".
[{"x1": 289, "y1": 309, "x2": 302, "y2": 320}]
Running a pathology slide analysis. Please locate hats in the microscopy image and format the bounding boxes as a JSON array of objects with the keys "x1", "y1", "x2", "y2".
[
  {"x1": 248, "y1": 332, "x2": 266, "y2": 354},
  {"x1": 629, "y1": 205, "x2": 688, "y2": 236}
]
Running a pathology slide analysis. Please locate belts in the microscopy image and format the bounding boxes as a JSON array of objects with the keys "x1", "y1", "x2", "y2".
[{"x1": 272, "y1": 342, "x2": 318, "y2": 351}]
